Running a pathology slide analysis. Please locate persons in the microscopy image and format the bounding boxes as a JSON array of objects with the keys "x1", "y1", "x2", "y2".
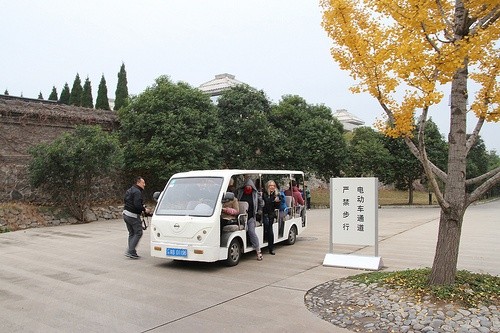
[
  {"x1": 171, "y1": 176, "x2": 288, "y2": 255},
  {"x1": 283, "y1": 180, "x2": 305, "y2": 227},
  {"x1": 234, "y1": 180, "x2": 263, "y2": 261},
  {"x1": 303, "y1": 185, "x2": 311, "y2": 211},
  {"x1": 123, "y1": 177, "x2": 150, "y2": 258}
]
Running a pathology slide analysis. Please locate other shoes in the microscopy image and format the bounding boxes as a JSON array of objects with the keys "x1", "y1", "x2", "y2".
[
  {"x1": 125, "y1": 252, "x2": 140, "y2": 258},
  {"x1": 257, "y1": 252, "x2": 263, "y2": 260},
  {"x1": 269, "y1": 250, "x2": 275, "y2": 255}
]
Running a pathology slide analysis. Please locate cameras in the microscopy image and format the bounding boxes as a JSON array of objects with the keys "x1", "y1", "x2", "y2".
[{"x1": 144, "y1": 211, "x2": 153, "y2": 217}]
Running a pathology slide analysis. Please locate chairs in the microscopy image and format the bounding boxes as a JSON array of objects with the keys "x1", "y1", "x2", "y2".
[{"x1": 222, "y1": 192, "x2": 296, "y2": 232}]
